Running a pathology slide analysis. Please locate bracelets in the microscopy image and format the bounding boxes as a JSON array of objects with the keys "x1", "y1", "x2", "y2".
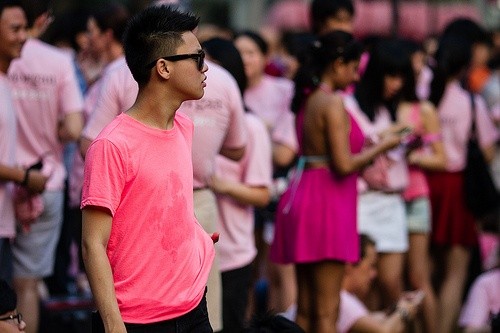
[{"x1": 22, "y1": 169, "x2": 30, "y2": 189}]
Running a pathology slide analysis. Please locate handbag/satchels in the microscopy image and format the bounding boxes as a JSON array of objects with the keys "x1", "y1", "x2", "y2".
[{"x1": 459, "y1": 92, "x2": 500, "y2": 233}]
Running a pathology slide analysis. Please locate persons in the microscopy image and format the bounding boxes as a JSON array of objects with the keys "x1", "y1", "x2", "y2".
[
  {"x1": 0, "y1": 0, "x2": 139, "y2": 333},
  {"x1": 80, "y1": 3, "x2": 220, "y2": 333},
  {"x1": 175, "y1": 0, "x2": 500, "y2": 333}
]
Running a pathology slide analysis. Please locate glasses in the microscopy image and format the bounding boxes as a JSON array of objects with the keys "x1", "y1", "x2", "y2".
[
  {"x1": 0, "y1": 312, "x2": 22, "y2": 323},
  {"x1": 144, "y1": 49, "x2": 205, "y2": 70}
]
